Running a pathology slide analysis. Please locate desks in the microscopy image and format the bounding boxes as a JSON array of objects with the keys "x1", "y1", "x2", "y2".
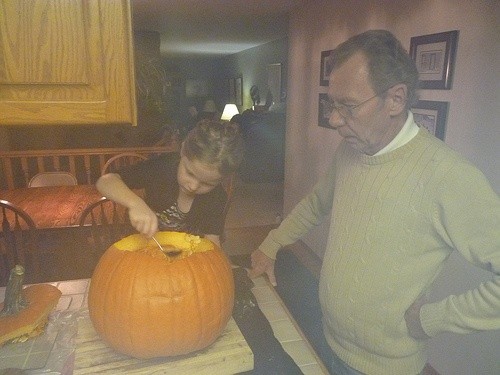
[{"x1": 0, "y1": 184, "x2": 145, "y2": 234}]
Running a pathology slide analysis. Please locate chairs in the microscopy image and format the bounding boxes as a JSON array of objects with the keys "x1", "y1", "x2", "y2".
[
  {"x1": 101, "y1": 152, "x2": 142, "y2": 176},
  {"x1": 28, "y1": 172, "x2": 78, "y2": 189},
  {"x1": 77, "y1": 197, "x2": 141, "y2": 280},
  {"x1": 0, "y1": 200, "x2": 60, "y2": 286}
]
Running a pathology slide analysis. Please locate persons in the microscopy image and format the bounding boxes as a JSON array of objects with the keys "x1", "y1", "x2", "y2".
[
  {"x1": 247, "y1": 30, "x2": 500, "y2": 375},
  {"x1": 96, "y1": 119, "x2": 245, "y2": 270}
]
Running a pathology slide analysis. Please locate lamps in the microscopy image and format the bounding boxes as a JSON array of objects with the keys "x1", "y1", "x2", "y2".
[
  {"x1": 202, "y1": 100, "x2": 218, "y2": 116},
  {"x1": 221, "y1": 103, "x2": 239, "y2": 122}
]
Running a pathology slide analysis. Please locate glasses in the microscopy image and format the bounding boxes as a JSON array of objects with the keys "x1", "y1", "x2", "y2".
[{"x1": 323, "y1": 82, "x2": 402, "y2": 119}]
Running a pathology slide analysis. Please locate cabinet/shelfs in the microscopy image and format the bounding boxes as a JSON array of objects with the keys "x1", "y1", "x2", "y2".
[{"x1": 0, "y1": 0, "x2": 137, "y2": 127}]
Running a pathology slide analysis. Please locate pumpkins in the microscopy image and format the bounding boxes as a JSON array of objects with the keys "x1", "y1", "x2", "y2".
[
  {"x1": 0, "y1": 264, "x2": 62, "y2": 347},
  {"x1": 89, "y1": 229, "x2": 234, "y2": 358}
]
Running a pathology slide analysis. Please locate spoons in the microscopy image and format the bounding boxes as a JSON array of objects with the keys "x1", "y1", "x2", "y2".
[{"x1": 153, "y1": 235, "x2": 182, "y2": 256}]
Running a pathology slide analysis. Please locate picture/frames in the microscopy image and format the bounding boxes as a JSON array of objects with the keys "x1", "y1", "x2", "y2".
[
  {"x1": 218, "y1": 75, "x2": 242, "y2": 105},
  {"x1": 318, "y1": 93, "x2": 337, "y2": 130},
  {"x1": 265, "y1": 63, "x2": 282, "y2": 101},
  {"x1": 411, "y1": 100, "x2": 448, "y2": 142},
  {"x1": 409, "y1": 30, "x2": 459, "y2": 90},
  {"x1": 319, "y1": 50, "x2": 334, "y2": 86}
]
